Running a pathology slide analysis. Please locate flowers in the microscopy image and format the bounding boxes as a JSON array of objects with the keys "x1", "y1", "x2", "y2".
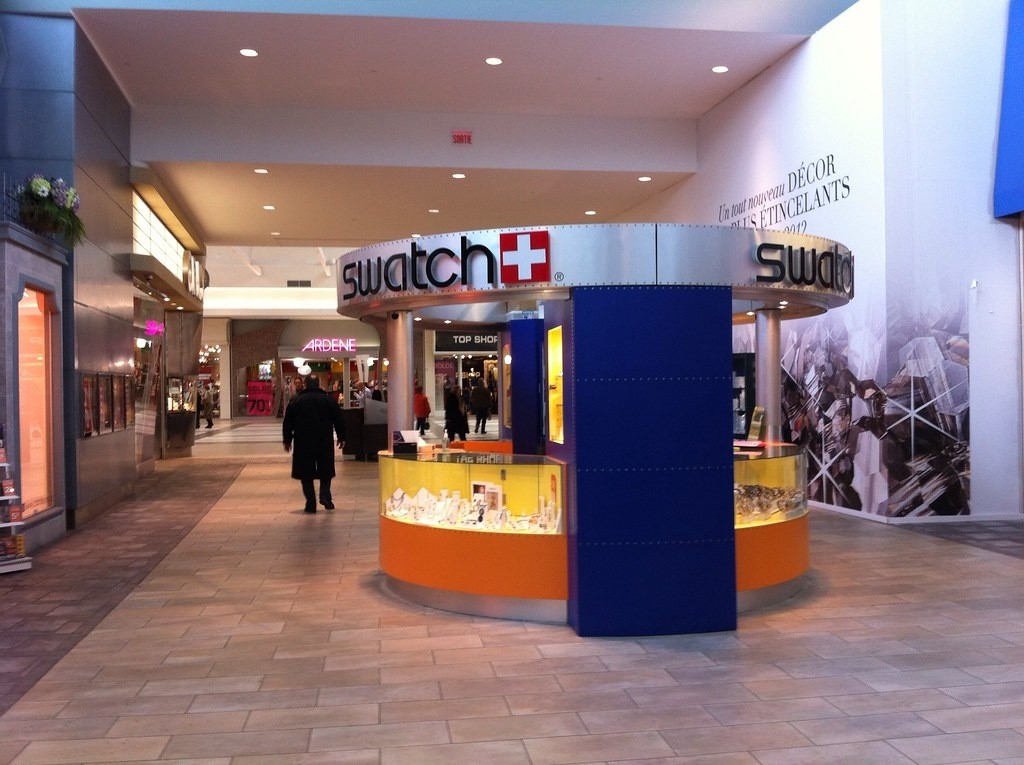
[{"x1": 15, "y1": 174, "x2": 80, "y2": 214}]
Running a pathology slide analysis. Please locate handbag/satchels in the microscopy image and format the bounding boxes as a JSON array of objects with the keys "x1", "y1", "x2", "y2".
[{"x1": 425, "y1": 416, "x2": 430, "y2": 430}]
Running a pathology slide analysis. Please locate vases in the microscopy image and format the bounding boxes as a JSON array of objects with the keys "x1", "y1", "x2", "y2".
[{"x1": 18, "y1": 205, "x2": 59, "y2": 236}]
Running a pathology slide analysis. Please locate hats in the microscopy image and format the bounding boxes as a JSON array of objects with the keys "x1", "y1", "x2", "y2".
[{"x1": 454, "y1": 385, "x2": 460, "y2": 392}]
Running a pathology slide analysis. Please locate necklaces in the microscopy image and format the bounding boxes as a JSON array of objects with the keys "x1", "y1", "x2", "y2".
[{"x1": 391, "y1": 491, "x2": 461, "y2": 524}]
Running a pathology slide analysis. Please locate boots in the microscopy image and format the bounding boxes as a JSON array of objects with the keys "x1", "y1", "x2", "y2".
[{"x1": 205, "y1": 418, "x2": 214, "y2": 428}]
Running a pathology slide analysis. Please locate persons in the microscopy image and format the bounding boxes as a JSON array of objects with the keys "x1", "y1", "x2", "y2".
[
  {"x1": 471, "y1": 377, "x2": 491, "y2": 434},
  {"x1": 196, "y1": 385, "x2": 214, "y2": 428},
  {"x1": 284, "y1": 378, "x2": 293, "y2": 393},
  {"x1": 282, "y1": 375, "x2": 347, "y2": 512},
  {"x1": 291, "y1": 377, "x2": 306, "y2": 400},
  {"x1": 330, "y1": 378, "x2": 388, "y2": 407},
  {"x1": 414, "y1": 374, "x2": 470, "y2": 443}
]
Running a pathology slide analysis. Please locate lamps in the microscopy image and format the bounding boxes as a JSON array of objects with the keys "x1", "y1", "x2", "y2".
[
  {"x1": 198, "y1": 343, "x2": 222, "y2": 364},
  {"x1": 136, "y1": 338, "x2": 147, "y2": 349},
  {"x1": 293, "y1": 356, "x2": 312, "y2": 376},
  {"x1": 504, "y1": 355, "x2": 513, "y2": 365}
]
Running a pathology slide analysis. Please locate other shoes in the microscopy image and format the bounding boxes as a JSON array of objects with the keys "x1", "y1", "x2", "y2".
[
  {"x1": 482, "y1": 431, "x2": 488, "y2": 434},
  {"x1": 420, "y1": 433, "x2": 425, "y2": 436},
  {"x1": 305, "y1": 506, "x2": 316, "y2": 513},
  {"x1": 320, "y1": 497, "x2": 334, "y2": 509},
  {"x1": 475, "y1": 428, "x2": 479, "y2": 433}
]
